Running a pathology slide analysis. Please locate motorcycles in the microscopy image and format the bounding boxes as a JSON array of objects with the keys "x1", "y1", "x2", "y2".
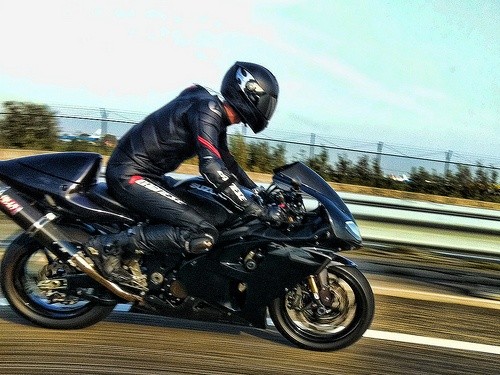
[{"x1": 0, "y1": 152, "x2": 376, "y2": 351}]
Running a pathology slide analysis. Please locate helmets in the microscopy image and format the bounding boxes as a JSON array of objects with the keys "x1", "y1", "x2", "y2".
[{"x1": 220, "y1": 61, "x2": 280, "y2": 134}]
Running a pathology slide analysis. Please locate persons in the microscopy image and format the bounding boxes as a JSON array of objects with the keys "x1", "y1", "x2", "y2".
[{"x1": 82, "y1": 60, "x2": 291, "y2": 285}]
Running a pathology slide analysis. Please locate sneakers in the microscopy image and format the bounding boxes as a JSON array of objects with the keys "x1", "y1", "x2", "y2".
[{"x1": 83, "y1": 233, "x2": 145, "y2": 293}]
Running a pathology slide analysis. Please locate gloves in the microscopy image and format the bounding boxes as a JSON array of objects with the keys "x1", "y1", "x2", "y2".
[
  {"x1": 258, "y1": 189, "x2": 279, "y2": 204},
  {"x1": 246, "y1": 203, "x2": 287, "y2": 228}
]
